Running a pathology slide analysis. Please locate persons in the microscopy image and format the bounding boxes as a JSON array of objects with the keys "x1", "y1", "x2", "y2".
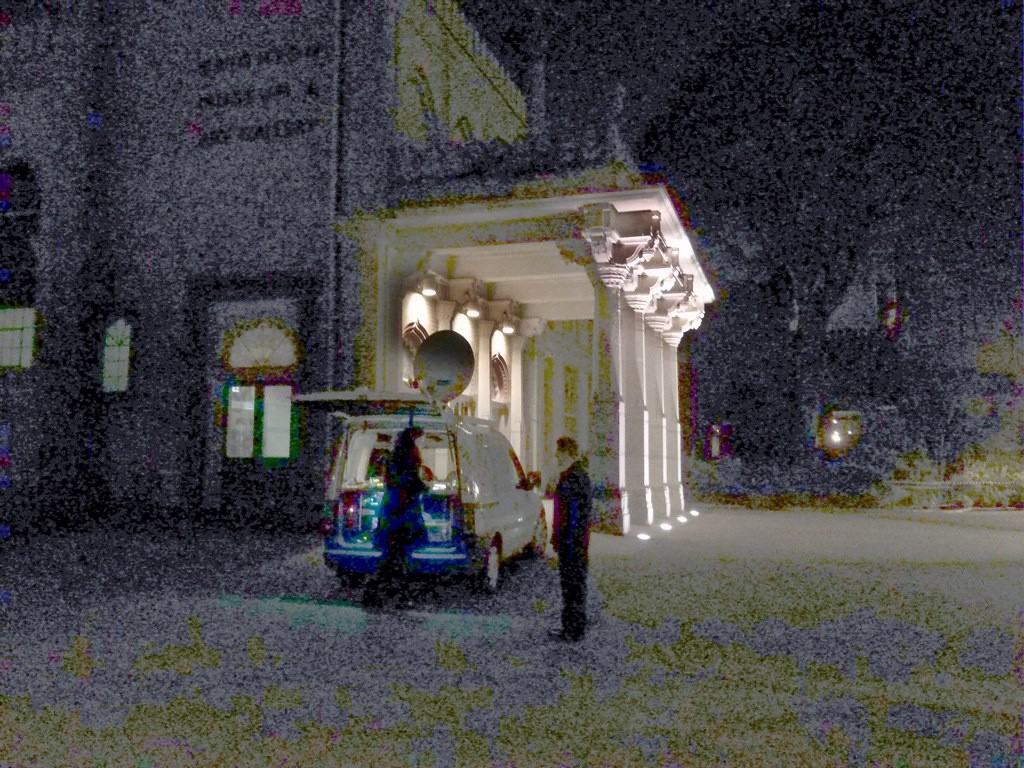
[
  {"x1": 548, "y1": 436, "x2": 593, "y2": 642},
  {"x1": 362, "y1": 426, "x2": 433, "y2": 610}
]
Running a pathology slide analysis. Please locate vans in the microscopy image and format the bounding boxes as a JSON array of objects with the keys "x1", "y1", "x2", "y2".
[{"x1": 288, "y1": 389, "x2": 547, "y2": 591}]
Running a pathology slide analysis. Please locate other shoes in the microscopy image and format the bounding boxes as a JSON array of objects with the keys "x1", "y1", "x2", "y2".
[{"x1": 547, "y1": 625, "x2": 581, "y2": 641}]
